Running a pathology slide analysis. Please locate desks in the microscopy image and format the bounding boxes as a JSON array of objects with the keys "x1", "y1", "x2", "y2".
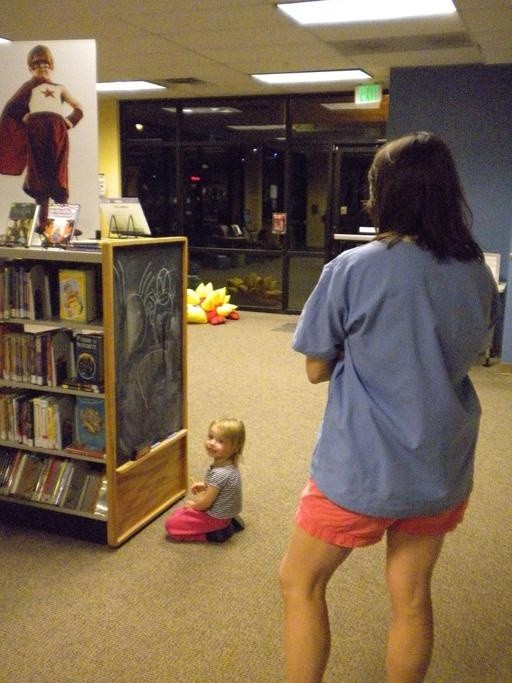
[{"x1": 481, "y1": 283, "x2": 507, "y2": 368}]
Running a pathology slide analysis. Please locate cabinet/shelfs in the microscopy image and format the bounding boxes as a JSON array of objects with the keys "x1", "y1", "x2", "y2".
[{"x1": 0, "y1": 237, "x2": 106, "y2": 526}]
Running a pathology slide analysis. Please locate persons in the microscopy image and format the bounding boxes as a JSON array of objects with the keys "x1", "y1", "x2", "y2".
[
  {"x1": 164, "y1": 416, "x2": 247, "y2": 543},
  {"x1": 0, "y1": 45, "x2": 86, "y2": 237},
  {"x1": 40, "y1": 218, "x2": 55, "y2": 243},
  {"x1": 59, "y1": 219, "x2": 75, "y2": 243},
  {"x1": 277, "y1": 133, "x2": 501, "y2": 683}
]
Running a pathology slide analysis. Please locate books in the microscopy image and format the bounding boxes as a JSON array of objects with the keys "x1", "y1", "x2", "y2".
[
  {"x1": 0, "y1": 259, "x2": 107, "y2": 518},
  {"x1": 4, "y1": 202, "x2": 39, "y2": 247},
  {"x1": 39, "y1": 202, "x2": 81, "y2": 247}
]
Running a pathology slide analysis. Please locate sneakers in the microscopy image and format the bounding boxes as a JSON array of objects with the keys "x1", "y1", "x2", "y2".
[
  {"x1": 207, "y1": 523, "x2": 233, "y2": 543},
  {"x1": 229, "y1": 514, "x2": 244, "y2": 533}
]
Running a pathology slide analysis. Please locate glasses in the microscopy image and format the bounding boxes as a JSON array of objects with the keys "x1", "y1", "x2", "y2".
[{"x1": 30, "y1": 58, "x2": 52, "y2": 65}]
